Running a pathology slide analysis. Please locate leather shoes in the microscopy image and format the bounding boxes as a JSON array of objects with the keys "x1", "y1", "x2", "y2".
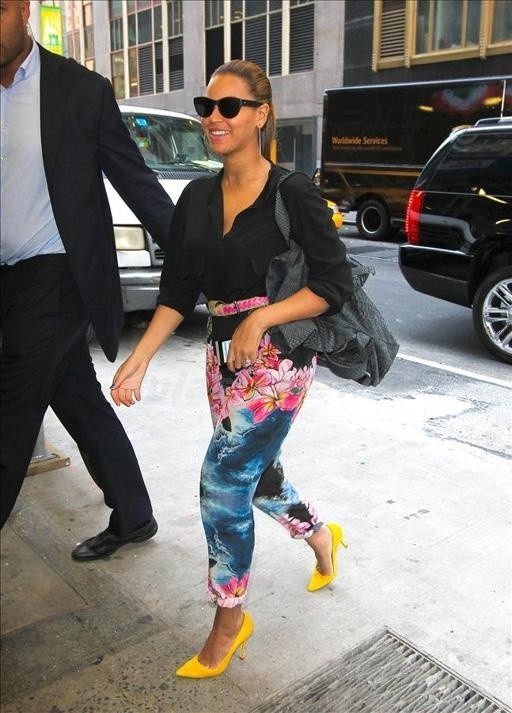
[{"x1": 72, "y1": 515, "x2": 158, "y2": 560}]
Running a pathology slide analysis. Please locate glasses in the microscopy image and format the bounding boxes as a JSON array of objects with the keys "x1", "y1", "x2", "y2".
[{"x1": 194, "y1": 96, "x2": 262, "y2": 118}]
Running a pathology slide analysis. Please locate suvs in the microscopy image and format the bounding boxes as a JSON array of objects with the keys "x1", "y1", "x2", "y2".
[{"x1": 394, "y1": 116, "x2": 511, "y2": 369}]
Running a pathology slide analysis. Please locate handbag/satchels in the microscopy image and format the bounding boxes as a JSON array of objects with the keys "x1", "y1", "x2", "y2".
[{"x1": 268, "y1": 248, "x2": 398, "y2": 385}]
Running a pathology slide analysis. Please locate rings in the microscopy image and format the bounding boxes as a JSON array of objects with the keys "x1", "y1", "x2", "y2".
[{"x1": 242, "y1": 359, "x2": 250, "y2": 366}]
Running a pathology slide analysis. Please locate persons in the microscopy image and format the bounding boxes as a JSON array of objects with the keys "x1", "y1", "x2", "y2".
[
  {"x1": 109, "y1": 57, "x2": 352, "y2": 683},
  {"x1": 1, "y1": 0, "x2": 177, "y2": 561}
]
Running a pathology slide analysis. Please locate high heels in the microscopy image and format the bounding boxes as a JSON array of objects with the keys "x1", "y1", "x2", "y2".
[
  {"x1": 308, "y1": 525, "x2": 347, "y2": 593},
  {"x1": 176, "y1": 611, "x2": 252, "y2": 679}
]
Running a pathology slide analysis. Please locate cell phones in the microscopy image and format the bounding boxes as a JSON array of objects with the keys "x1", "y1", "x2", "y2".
[{"x1": 219, "y1": 337, "x2": 232, "y2": 366}]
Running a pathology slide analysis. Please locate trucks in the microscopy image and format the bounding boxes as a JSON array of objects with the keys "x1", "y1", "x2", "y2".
[{"x1": 311, "y1": 74, "x2": 512, "y2": 241}]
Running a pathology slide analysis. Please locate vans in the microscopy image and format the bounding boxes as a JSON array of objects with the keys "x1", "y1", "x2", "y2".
[{"x1": 100, "y1": 106, "x2": 227, "y2": 321}]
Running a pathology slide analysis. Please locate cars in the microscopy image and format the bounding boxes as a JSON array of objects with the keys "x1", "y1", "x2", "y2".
[{"x1": 320, "y1": 192, "x2": 345, "y2": 232}]
